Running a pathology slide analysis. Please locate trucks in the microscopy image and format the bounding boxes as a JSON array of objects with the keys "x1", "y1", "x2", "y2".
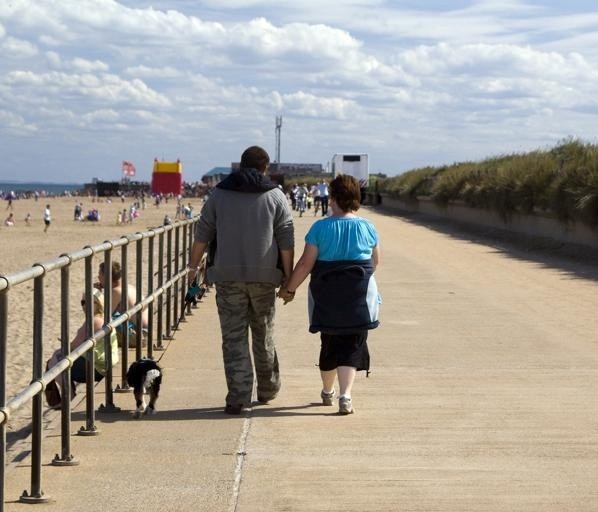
[{"x1": 331, "y1": 152, "x2": 369, "y2": 203}]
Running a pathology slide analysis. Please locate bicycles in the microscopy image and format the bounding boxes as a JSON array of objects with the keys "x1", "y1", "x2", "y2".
[
  {"x1": 295, "y1": 197, "x2": 305, "y2": 218},
  {"x1": 313, "y1": 201, "x2": 320, "y2": 215}
]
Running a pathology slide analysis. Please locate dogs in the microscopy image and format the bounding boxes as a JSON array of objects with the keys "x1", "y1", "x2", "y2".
[{"x1": 126, "y1": 360, "x2": 164, "y2": 419}]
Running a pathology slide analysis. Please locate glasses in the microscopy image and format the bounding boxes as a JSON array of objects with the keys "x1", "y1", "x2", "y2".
[{"x1": 81, "y1": 300, "x2": 97, "y2": 306}]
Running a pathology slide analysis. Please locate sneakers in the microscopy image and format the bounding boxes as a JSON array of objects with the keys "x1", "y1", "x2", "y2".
[
  {"x1": 321, "y1": 390, "x2": 335, "y2": 406},
  {"x1": 339, "y1": 396, "x2": 355, "y2": 413},
  {"x1": 224, "y1": 404, "x2": 243, "y2": 414}
]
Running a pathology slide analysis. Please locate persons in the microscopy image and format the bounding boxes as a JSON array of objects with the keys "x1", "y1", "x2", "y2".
[
  {"x1": 93, "y1": 259, "x2": 153, "y2": 350},
  {"x1": 291, "y1": 180, "x2": 330, "y2": 217},
  {"x1": 4, "y1": 188, "x2": 100, "y2": 233},
  {"x1": 282, "y1": 174, "x2": 380, "y2": 414},
  {"x1": 48, "y1": 287, "x2": 120, "y2": 400},
  {"x1": 186, "y1": 146, "x2": 295, "y2": 413},
  {"x1": 105, "y1": 188, "x2": 193, "y2": 225}
]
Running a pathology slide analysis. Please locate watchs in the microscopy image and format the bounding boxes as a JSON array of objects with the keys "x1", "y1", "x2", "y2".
[{"x1": 186, "y1": 263, "x2": 198, "y2": 272}]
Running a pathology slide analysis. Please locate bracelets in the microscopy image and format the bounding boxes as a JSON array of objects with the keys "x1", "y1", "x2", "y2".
[{"x1": 285, "y1": 288, "x2": 296, "y2": 295}]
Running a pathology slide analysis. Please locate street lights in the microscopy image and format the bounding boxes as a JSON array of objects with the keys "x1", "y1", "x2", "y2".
[{"x1": 274, "y1": 113, "x2": 282, "y2": 162}]
